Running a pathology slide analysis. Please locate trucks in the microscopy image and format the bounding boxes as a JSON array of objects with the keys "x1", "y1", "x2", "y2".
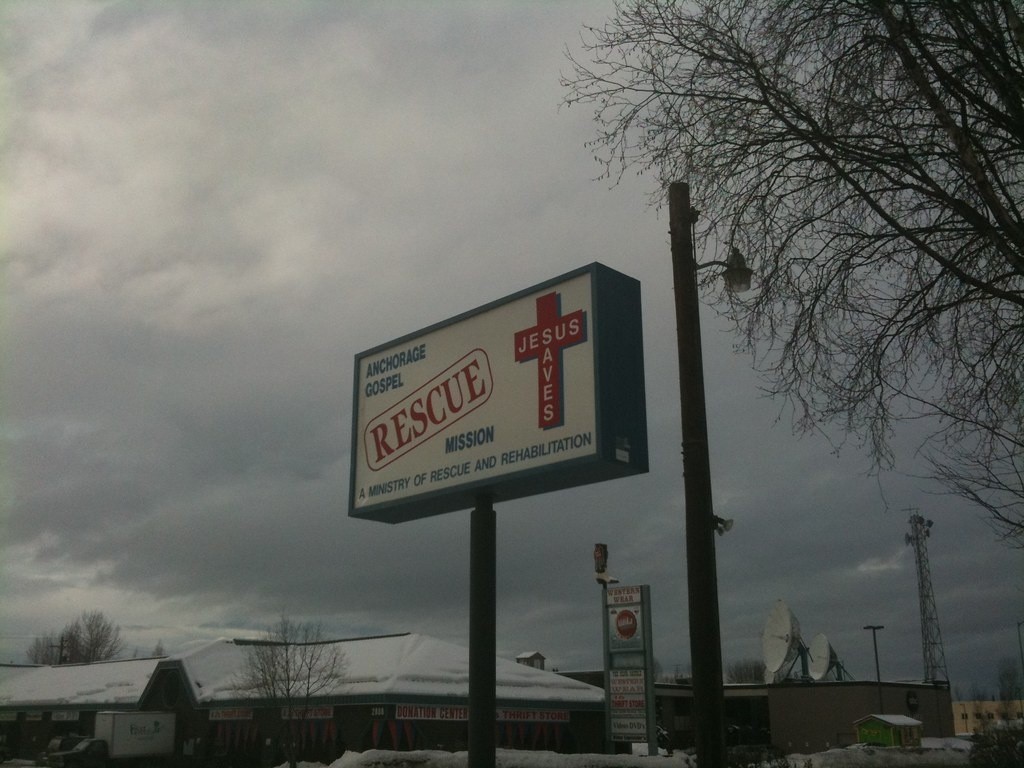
[{"x1": 49, "y1": 712, "x2": 176, "y2": 767}]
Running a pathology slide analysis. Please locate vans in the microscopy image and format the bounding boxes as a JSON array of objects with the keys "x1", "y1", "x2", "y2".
[
  {"x1": 0, "y1": 731, "x2": 16, "y2": 763},
  {"x1": 48, "y1": 736, "x2": 86, "y2": 753}
]
